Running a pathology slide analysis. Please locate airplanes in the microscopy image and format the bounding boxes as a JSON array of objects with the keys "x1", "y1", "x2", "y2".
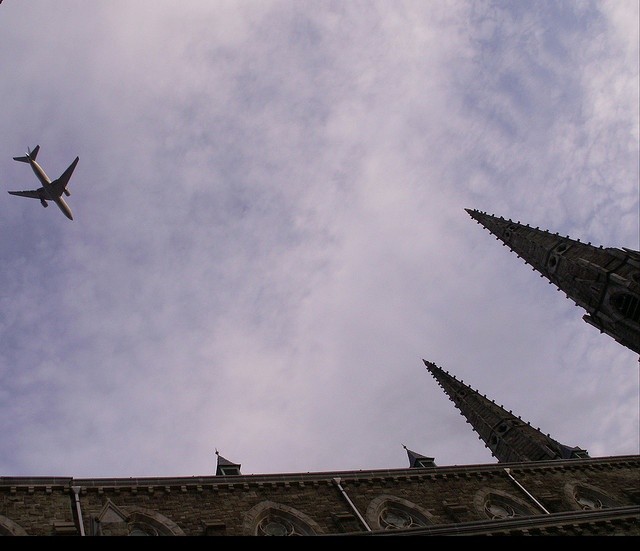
[{"x1": 7, "y1": 145, "x2": 79, "y2": 221}]
